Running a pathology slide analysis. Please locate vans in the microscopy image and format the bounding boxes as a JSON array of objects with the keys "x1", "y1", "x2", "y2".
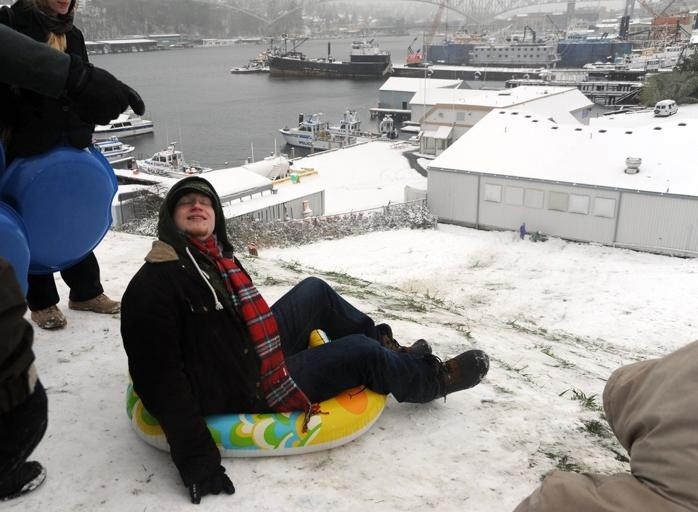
[{"x1": 654, "y1": 99, "x2": 678, "y2": 116}]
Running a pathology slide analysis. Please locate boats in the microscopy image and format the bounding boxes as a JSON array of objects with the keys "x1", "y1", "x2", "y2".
[
  {"x1": 94, "y1": 136, "x2": 135, "y2": 166},
  {"x1": 279, "y1": 107, "x2": 380, "y2": 151},
  {"x1": 91, "y1": 109, "x2": 154, "y2": 139},
  {"x1": 231, "y1": 33, "x2": 391, "y2": 79},
  {"x1": 135, "y1": 141, "x2": 213, "y2": 180}
]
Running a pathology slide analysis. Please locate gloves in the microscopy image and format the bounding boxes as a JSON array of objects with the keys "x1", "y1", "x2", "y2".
[
  {"x1": 65, "y1": 53, "x2": 146, "y2": 126},
  {"x1": 189, "y1": 465, "x2": 235, "y2": 505}
]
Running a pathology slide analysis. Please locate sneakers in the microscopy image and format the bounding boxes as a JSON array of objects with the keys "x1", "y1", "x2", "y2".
[
  {"x1": 1, "y1": 460, "x2": 47, "y2": 500},
  {"x1": 31, "y1": 304, "x2": 66, "y2": 329},
  {"x1": 376, "y1": 323, "x2": 432, "y2": 354},
  {"x1": 423, "y1": 349, "x2": 490, "y2": 403},
  {"x1": 69, "y1": 294, "x2": 121, "y2": 314}
]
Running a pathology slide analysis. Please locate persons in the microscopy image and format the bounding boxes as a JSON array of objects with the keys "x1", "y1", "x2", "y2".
[
  {"x1": 520, "y1": 222, "x2": 527, "y2": 239},
  {"x1": 508, "y1": 334, "x2": 698, "y2": 512},
  {"x1": 0, "y1": 0, "x2": 124, "y2": 332},
  {"x1": 1, "y1": 22, "x2": 147, "y2": 503},
  {"x1": 119, "y1": 175, "x2": 491, "y2": 505}
]
